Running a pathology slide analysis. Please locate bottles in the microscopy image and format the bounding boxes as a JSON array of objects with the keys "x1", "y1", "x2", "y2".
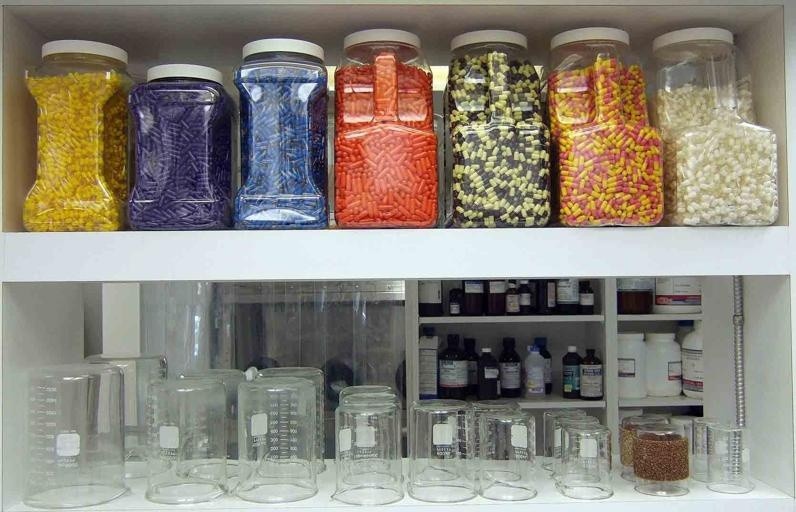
[
  {"x1": 578, "y1": 281, "x2": 595, "y2": 315},
  {"x1": 616, "y1": 320, "x2": 704, "y2": 401},
  {"x1": 497, "y1": 336, "x2": 523, "y2": 398},
  {"x1": 518, "y1": 280, "x2": 532, "y2": 314},
  {"x1": 448, "y1": 288, "x2": 460, "y2": 316},
  {"x1": 418, "y1": 280, "x2": 444, "y2": 317},
  {"x1": 461, "y1": 337, "x2": 482, "y2": 397},
  {"x1": 562, "y1": 345, "x2": 584, "y2": 399},
  {"x1": 485, "y1": 281, "x2": 506, "y2": 316},
  {"x1": 461, "y1": 280, "x2": 485, "y2": 315},
  {"x1": 478, "y1": 348, "x2": 502, "y2": 399},
  {"x1": 536, "y1": 280, "x2": 558, "y2": 315},
  {"x1": 578, "y1": 348, "x2": 604, "y2": 401},
  {"x1": 555, "y1": 278, "x2": 580, "y2": 315},
  {"x1": 505, "y1": 280, "x2": 521, "y2": 315},
  {"x1": 438, "y1": 334, "x2": 470, "y2": 401},
  {"x1": 523, "y1": 337, "x2": 551, "y2": 400}
]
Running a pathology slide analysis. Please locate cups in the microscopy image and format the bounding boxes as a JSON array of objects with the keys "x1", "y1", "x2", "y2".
[
  {"x1": 330, "y1": 386, "x2": 405, "y2": 503},
  {"x1": 691, "y1": 418, "x2": 757, "y2": 494},
  {"x1": 465, "y1": 400, "x2": 538, "y2": 501},
  {"x1": 145, "y1": 370, "x2": 244, "y2": 505},
  {"x1": 236, "y1": 366, "x2": 327, "y2": 504},
  {"x1": 542, "y1": 409, "x2": 613, "y2": 500},
  {"x1": 85, "y1": 353, "x2": 174, "y2": 480},
  {"x1": 406, "y1": 400, "x2": 482, "y2": 502},
  {"x1": 21, "y1": 361, "x2": 129, "y2": 507}
]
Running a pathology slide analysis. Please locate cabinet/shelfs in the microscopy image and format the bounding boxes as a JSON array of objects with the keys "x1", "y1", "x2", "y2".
[
  {"x1": 410, "y1": 278, "x2": 609, "y2": 460},
  {"x1": 611, "y1": 278, "x2": 705, "y2": 410},
  {"x1": 2, "y1": 0, "x2": 792, "y2": 510}
]
